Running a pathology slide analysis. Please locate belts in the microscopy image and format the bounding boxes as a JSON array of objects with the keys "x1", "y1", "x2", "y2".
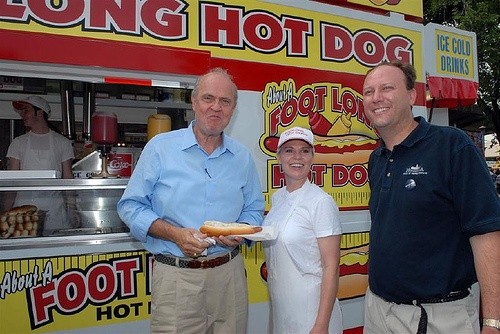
[
  {"x1": 368, "y1": 284, "x2": 475, "y2": 331},
  {"x1": 153, "y1": 244, "x2": 240, "y2": 270}
]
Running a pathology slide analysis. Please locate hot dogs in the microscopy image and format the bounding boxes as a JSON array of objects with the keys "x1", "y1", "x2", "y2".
[
  {"x1": 261, "y1": 243, "x2": 370, "y2": 301},
  {"x1": 200, "y1": 220, "x2": 262, "y2": 237},
  {"x1": 264, "y1": 134, "x2": 380, "y2": 168},
  {"x1": 0, "y1": 205, "x2": 39, "y2": 238}
]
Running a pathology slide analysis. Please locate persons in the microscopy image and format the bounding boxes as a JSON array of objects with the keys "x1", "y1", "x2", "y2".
[
  {"x1": 262, "y1": 128, "x2": 344, "y2": 333},
  {"x1": 118, "y1": 70, "x2": 264, "y2": 334},
  {"x1": 363, "y1": 60, "x2": 500, "y2": 333},
  {"x1": 1, "y1": 96, "x2": 81, "y2": 233}
]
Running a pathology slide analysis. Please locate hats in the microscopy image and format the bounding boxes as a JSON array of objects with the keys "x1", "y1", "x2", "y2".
[
  {"x1": 277, "y1": 126, "x2": 314, "y2": 150},
  {"x1": 11, "y1": 95, "x2": 53, "y2": 119}
]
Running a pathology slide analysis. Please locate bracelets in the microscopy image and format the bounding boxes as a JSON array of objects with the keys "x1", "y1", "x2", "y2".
[{"x1": 482, "y1": 318, "x2": 500, "y2": 329}]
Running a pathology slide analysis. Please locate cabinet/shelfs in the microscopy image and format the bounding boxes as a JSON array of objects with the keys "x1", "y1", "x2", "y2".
[{"x1": 0, "y1": 179, "x2": 138, "y2": 251}]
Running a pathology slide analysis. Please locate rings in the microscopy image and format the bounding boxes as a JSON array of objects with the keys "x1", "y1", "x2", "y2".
[{"x1": 193, "y1": 253, "x2": 198, "y2": 259}]
